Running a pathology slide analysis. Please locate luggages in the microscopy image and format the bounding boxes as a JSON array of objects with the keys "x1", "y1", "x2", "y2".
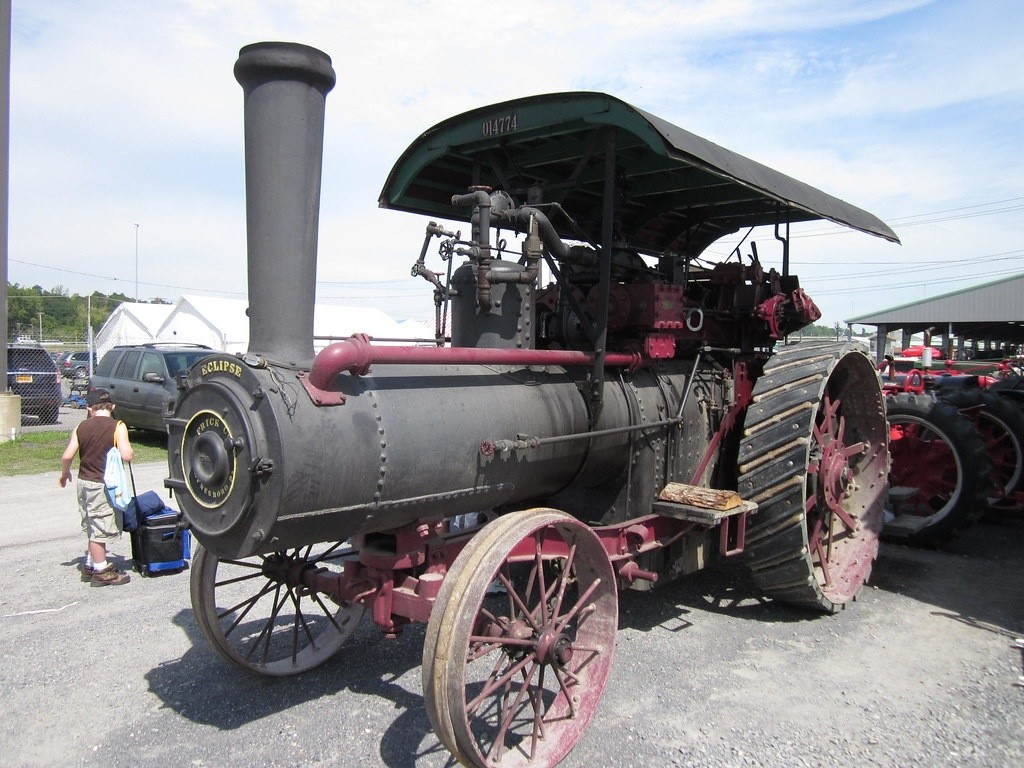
[{"x1": 128, "y1": 460, "x2": 192, "y2": 577}]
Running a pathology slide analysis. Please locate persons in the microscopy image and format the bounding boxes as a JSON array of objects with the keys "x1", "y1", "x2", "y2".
[{"x1": 59, "y1": 389, "x2": 135, "y2": 587}]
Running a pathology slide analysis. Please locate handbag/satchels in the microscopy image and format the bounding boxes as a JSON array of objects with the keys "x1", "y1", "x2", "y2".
[{"x1": 122, "y1": 489, "x2": 165, "y2": 532}]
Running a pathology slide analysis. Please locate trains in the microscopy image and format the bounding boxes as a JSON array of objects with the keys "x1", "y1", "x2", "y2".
[{"x1": 156, "y1": 27, "x2": 902, "y2": 766}]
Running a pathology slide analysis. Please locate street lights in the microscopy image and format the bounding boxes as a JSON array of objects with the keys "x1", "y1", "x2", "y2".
[{"x1": 132, "y1": 222, "x2": 141, "y2": 304}]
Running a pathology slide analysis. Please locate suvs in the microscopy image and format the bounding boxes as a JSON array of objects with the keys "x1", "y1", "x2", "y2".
[
  {"x1": 56, "y1": 350, "x2": 98, "y2": 379},
  {"x1": 86, "y1": 341, "x2": 221, "y2": 442},
  {"x1": 5, "y1": 342, "x2": 62, "y2": 425},
  {"x1": 14, "y1": 336, "x2": 38, "y2": 344}
]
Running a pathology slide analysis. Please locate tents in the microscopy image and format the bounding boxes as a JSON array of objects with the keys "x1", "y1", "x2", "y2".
[{"x1": 94, "y1": 295, "x2": 415, "y2": 367}]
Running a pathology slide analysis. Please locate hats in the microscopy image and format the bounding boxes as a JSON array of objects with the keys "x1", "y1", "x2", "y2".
[{"x1": 86, "y1": 388, "x2": 115, "y2": 407}]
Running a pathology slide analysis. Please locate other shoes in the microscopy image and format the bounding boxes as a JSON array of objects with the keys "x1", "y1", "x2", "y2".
[{"x1": 81, "y1": 561, "x2": 131, "y2": 587}]
